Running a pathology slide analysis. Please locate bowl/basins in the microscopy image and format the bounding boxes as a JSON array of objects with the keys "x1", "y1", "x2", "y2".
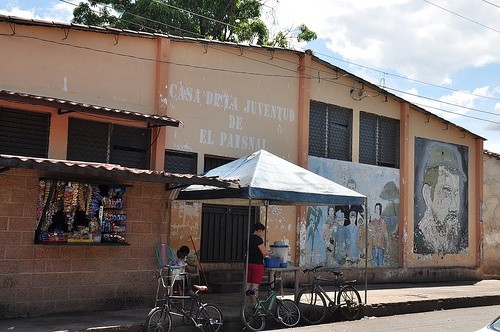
[{"x1": 264, "y1": 259, "x2": 282, "y2": 268}]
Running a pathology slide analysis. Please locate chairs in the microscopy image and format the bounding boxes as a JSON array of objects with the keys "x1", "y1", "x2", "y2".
[{"x1": 152, "y1": 243, "x2": 200, "y2": 313}]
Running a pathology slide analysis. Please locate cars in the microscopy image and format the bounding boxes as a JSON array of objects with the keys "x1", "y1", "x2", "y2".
[{"x1": 474, "y1": 315, "x2": 500, "y2": 332}]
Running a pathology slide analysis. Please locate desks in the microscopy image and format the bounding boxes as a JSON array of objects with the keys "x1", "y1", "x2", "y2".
[{"x1": 263, "y1": 266, "x2": 302, "y2": 301}]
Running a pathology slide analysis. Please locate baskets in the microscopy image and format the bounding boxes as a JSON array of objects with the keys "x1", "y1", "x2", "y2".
[{"x1": 264, "y1": 257, "x2": 281, "y2": 267}]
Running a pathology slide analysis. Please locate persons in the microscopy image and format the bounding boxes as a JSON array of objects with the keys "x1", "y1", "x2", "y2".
[{"x1": 245, "y1": 223, "x2": 269, "y2": 309}]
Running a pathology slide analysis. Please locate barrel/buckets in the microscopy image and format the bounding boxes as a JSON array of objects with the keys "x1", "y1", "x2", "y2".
[{"x1": 269, "y1": 245, "x2": 289, "y2": 268}]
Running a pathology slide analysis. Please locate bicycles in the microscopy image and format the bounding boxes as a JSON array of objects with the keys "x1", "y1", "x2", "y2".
[
  {"x1": 240, "y1": 272, "x2": 302, "y2": 331},
  {"x1": 296, "y1": 266, "x2": 362, "y2": 324},
  {"x1": 145, "y1": 272, "x2": 224, "y2": 332}
]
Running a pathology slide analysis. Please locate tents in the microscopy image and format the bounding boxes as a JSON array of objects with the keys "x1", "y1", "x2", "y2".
[{"x1": 173, "y1": 149, "x2": 368, "y2": 305}]
